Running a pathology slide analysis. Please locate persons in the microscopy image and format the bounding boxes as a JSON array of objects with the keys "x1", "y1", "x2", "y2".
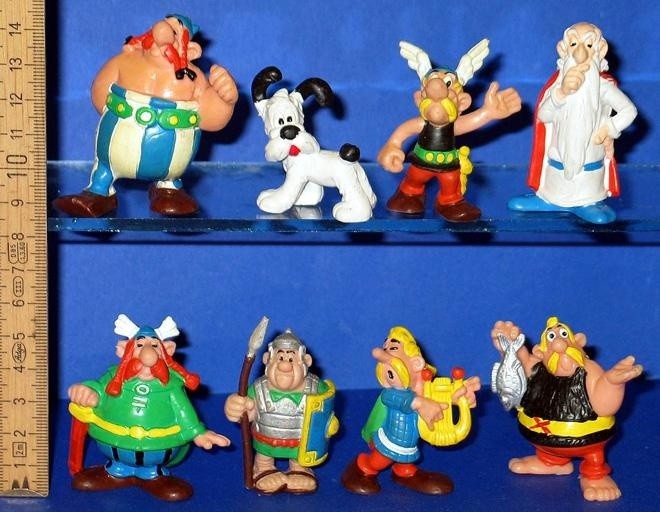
[
  {"x1": 51, "y1": 13, "x2": 240, "y2": 219},
  {"x1": 66, "y1": 312, "x2": 233, "y2": 503},
  {"x1": 376, "y1": 36, "x2": 524, "y2": 224},
  {"x1": 489, "y1": 314, "x2": 645, "y2": 503},
  {"x1": 507, "y1": 21, "x2": 639, "y2": 225},
  {"x1": 340, "y1": 323, "x2": 482, "y2": 496},
  {"x1": 222, "y1": 314, "x2": 341, "y2": 495}
]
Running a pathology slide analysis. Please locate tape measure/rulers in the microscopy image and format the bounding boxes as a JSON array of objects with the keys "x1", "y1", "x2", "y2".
[{"x1": 0, "y1": 0, "x2": 50, "y2": 497}]
